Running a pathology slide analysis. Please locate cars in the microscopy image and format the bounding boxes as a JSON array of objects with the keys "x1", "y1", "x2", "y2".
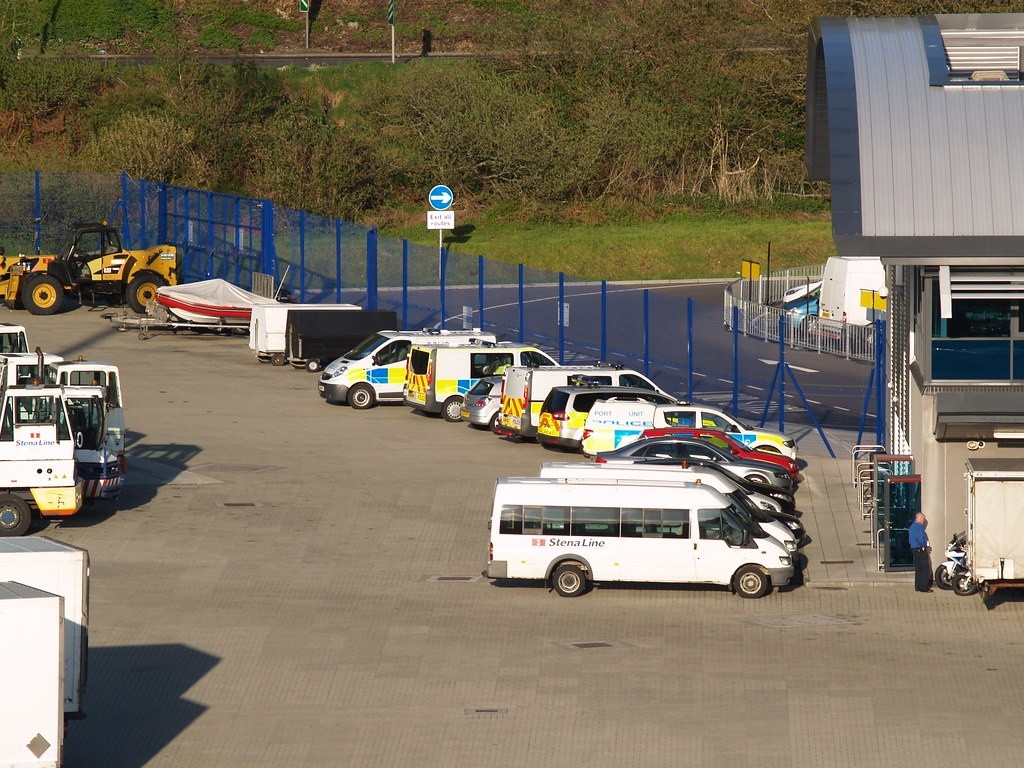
[
  {"x1": 761, "y1": 509, "x2": 807, "y2": 550},
  {"x1": 459, "y1": 375, "x2": 502, "y2": 432},
  {"x1": 594, "y1": 437, "x2": 796, "y2": 500},
  {"x1": 632, "y1": 428, "x2": 800, "y2": 484},
  {"x1": 632, "y1": 457, "x2": 797, "y2": 520}
]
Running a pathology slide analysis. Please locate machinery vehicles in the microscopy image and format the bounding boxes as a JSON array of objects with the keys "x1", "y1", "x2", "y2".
[{"x1": 0, "y1": 221, "x2": 188, "y2": 316}]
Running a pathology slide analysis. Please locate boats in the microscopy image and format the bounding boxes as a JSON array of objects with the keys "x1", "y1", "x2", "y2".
[{"x1": 151, "y1": 265, "x2": 291, "y2": 325}]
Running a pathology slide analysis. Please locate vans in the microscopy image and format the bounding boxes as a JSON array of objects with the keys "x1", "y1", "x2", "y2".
[
  {"x1": 495, "y1": 366, "x2": 677, "y2": 444},
  {"x1": 480, "y1": 473, "x2": 797, "y2": 599},
  {"x1": 541, "y1": 460, "x2": 799, "y2": 569},
  {"x1": 402, "y1": 338, "x2": 562, "y2": 422},
  {"x1": 698, "y1": 462, "x2": 784, "y2": 514},
  {"x1": 318, "y1": 327, "x2": 497, "y2": 410}
]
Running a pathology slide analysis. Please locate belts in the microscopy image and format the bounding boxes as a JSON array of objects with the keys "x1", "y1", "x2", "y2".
[{"x1": 912, "y1": 549, "x2": 926, "y2": 552}]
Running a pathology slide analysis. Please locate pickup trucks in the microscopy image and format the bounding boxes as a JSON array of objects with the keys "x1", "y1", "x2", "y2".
[{"x1": 581, "y1": 397, "x2": 797, "y2": 461}]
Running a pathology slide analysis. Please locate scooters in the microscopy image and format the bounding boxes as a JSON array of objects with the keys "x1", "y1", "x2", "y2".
[{"x1": 935, "y1": 531, "x2": 977, "y2": 597}]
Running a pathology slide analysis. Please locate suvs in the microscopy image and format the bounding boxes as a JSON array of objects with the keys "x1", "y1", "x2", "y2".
[
  {"x1": 537, "y1": 373, "x2": 724, "y2": 453},
  {"x1": 0, "y1": 322, "x2": 129, "y2": 539}
]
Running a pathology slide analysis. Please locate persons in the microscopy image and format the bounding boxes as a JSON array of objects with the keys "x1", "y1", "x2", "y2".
[{"x1": 908, "y1": 512, "x2": 934, "y2": 593}]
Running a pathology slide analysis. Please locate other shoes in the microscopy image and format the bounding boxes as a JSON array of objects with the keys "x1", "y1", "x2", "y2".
[
  {"x1": 920, "y1": 588, "x2": 933, "y2": 593},
  {"x1": 915, "y1": 587, "x2": 921, "y2": 591}
]
